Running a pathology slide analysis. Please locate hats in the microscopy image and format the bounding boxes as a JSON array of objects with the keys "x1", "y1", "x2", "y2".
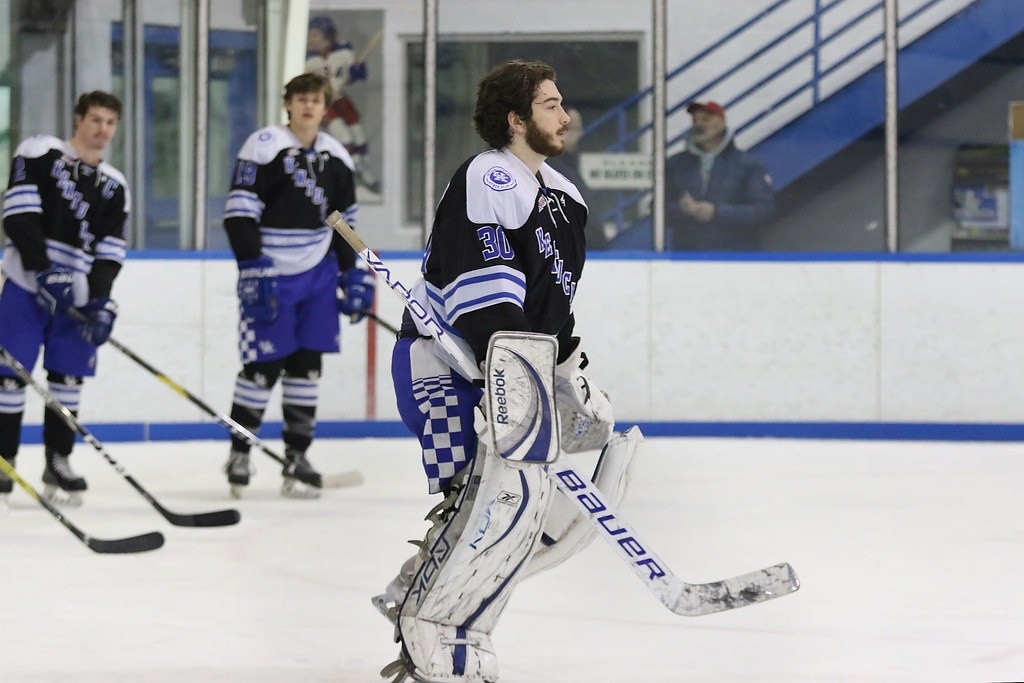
[{"x1": 687, "y1": 102, "x2": 724, "y2": 120}]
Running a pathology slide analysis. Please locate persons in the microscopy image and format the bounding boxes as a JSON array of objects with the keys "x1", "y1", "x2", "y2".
[
  {"x1": 547, "y1": 105, "x2": 604, "y2": 252},
  {"x1": 304, "y1": 17, "x2": 381, "y2": 192},
  {"x1": 664, "y1": 101, "x2": 774, "y2": 254},
  {"x1": 220, "y1": 71, "x2": 375, "y2": 497},
  {"x1": 0, "y1": 91, "x2": 131, "y2": 508},
  {"x1": 374, "y1": 57, "x2": 612, "y2": 683}
]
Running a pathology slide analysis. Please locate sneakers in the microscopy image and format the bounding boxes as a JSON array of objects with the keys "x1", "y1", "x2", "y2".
[
  {"x1": 281, "y1": 448, "x2": 321, "y2": 497},
  {"x1": 42, "y1": 449, "x2": 86, "y2": 507},
  {"x1": 356, "y1": 165, "x2": 380, "y2": 193},
  {"x1": 227, "y1": 451, "x2": 250, "y2": 497},
  {"x1": 0, "y1": 458, "x2": 15, "y2": 501}
]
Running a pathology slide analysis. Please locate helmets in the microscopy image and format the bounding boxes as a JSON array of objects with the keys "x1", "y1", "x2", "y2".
[{"x1": 309, "y1": 15, "x2": 335, "y2": 39}]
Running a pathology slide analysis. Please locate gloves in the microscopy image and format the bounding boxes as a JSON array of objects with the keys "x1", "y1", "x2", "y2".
[
  {"x1": 241, "y1": 256, "x2": 278, "y2": 316},
  {"x1": 340, "y1": 268, "x2": 373, "y2": 324},
  {"x1": 79, "y1": 296, "x2": 119, "y2": 345},
  {"x1": 36, "y1": 267, "x2": 75, "y2": 315},
  {"x1": 348, "y1": 64, "x2": 366, "y2": 79}
]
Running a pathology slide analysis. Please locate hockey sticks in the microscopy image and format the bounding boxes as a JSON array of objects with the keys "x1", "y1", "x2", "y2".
[
  {"x1": 0, "y1": 346, "x2": 243, "y2": 528},
  {"x1": 324, "y1": 206, "x2": 805, "y2": 619},
  {"x1": 66, "y1": 306, "x2": 365, "y2": 490},
  {"x1": 0, "y1": 455, "x2": 165, "y2": 554},
  {"x1": 365, "y1": 310, "x2": 403, "y2": 337}
]
